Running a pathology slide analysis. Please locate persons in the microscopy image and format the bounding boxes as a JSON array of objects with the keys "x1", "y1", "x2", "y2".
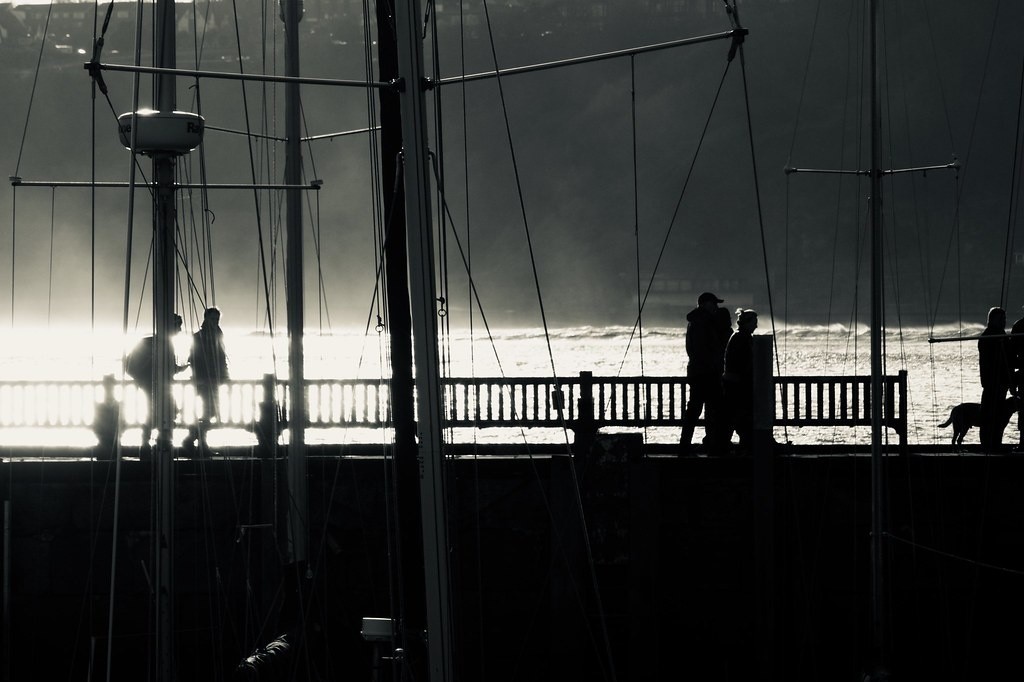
[
  {"x1": 978, "y1": 307, "x2": 1013, "y2": 453},
  {"x1": 139, "y1": 314, "x2": 189, "y2": 459},
  {"x1": 720, "y1": 308, "x2": 759, "y2": 448},
  {"x1": 679, "y1": 291, "x2": 729, "y2": 446},
  {"x1": 1009, "y1": 318, "x2": 1024, "y2": 450},
  {"x1": 182, "y1": 308, "x2": 233, "y2": 456}
]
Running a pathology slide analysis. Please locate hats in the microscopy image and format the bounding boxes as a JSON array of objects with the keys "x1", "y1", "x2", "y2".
[{"x1": 698, "y1": 292, "x2": 724, "y2": 305}]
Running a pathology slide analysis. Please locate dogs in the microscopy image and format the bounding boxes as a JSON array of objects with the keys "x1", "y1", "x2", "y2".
[{"x1": 938, "y1": 395, "x2": 1020, "y2": 449}]
